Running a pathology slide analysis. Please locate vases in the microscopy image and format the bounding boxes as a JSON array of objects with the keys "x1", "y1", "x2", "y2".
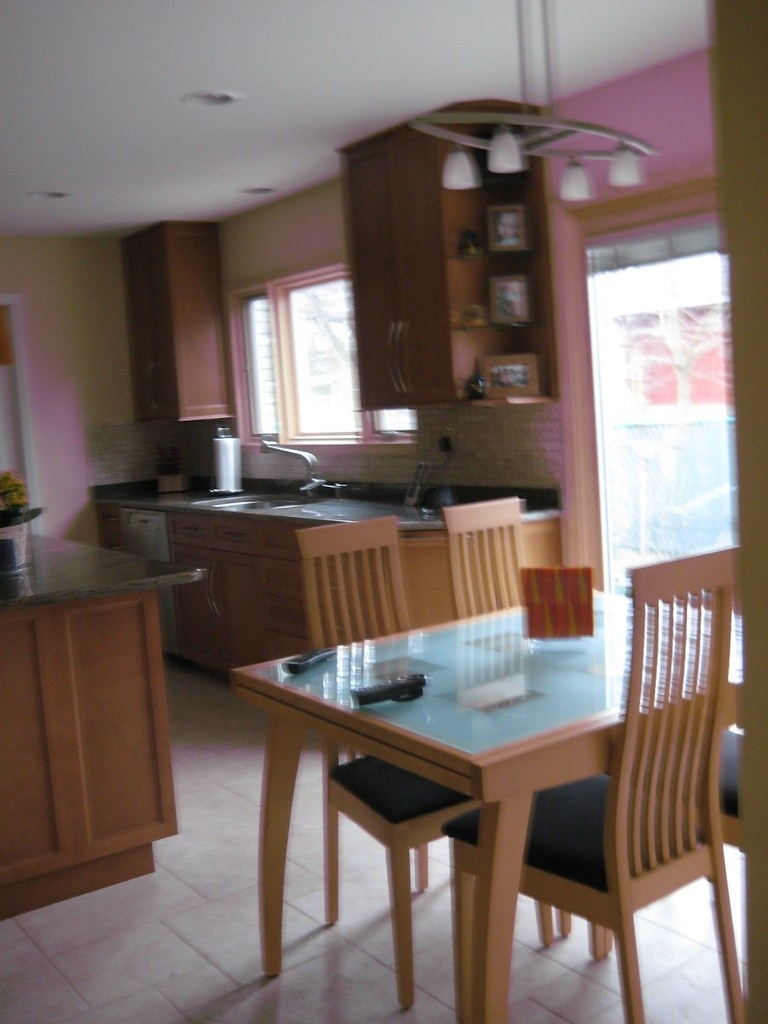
[{"x1": 0, "y1": 525, "x2": 30, "y2": 572}]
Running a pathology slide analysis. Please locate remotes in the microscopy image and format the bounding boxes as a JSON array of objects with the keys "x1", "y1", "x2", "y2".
[
  {"x1": 352, "y1": 673, "x2": 428, "y2": 705},
  {"x1": 285, "y1": 648, "x2": 337, "y2": 675}
]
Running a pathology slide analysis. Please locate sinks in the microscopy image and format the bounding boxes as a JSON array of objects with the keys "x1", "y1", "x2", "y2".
[{"x1": 208, "y1": 498, "x2": 302, "y2": 513}]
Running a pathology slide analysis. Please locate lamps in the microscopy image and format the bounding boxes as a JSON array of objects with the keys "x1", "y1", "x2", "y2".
[{"x1": 411, "y1": 2, "x2": 661, "y2": 204}]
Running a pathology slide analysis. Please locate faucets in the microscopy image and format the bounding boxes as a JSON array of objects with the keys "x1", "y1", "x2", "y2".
[{"x1": 259, "y1": 442, "x2": 328, "y2": 497}]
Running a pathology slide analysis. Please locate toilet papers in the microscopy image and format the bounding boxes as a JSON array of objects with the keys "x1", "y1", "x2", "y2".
[{"x1": 212, "y1": 438, "x2": 243, "y2": 490}]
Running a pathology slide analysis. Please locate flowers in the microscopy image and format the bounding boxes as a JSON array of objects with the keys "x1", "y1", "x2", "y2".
[{"x1": 0, "y1": 470, "x2": 43, "y2": 525}]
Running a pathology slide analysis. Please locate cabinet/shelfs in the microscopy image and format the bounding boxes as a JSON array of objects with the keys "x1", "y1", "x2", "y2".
[
  {"x1": 264, "y1": 515, "x2": 560, "y2": 658},
  {"x1": 93, "y1": 501, "x2": 120, "y2": 550},
  {"x1": 174, "y1": 505, "x2": 261, "y2": 689},
  {"x1": 337, "y1": 103, "x2": 562, "y2": 410},
  {"x1": 122, "y1": 218, "x2": 232, "y2": 420}
]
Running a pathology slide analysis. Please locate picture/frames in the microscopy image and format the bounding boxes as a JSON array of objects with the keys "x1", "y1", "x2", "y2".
[
  {"x1": 490, "y1": 276, "x2": 529, "y2": 326},
  {"x1": 477, "y1": 354, "x2": 541, "y2": 397},
  {"x1": 486, "y1": 207, "x2": 529, "y2": 248}
]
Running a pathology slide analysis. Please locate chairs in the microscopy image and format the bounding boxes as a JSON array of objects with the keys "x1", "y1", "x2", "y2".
[
  {"x1": 444, "y1": 495, "x2": 523, "y2": 618},
  {"x1": 521, "y1": 551, "x2": 742, "y2": 1024},
  {"x1": 292, "y1": 518, "x2": 475, "y2": 1011}
]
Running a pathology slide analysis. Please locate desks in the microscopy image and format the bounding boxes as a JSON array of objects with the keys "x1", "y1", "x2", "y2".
[{"x1": 228, "y1": 589, "x2": 746, "y2": 1024}]
